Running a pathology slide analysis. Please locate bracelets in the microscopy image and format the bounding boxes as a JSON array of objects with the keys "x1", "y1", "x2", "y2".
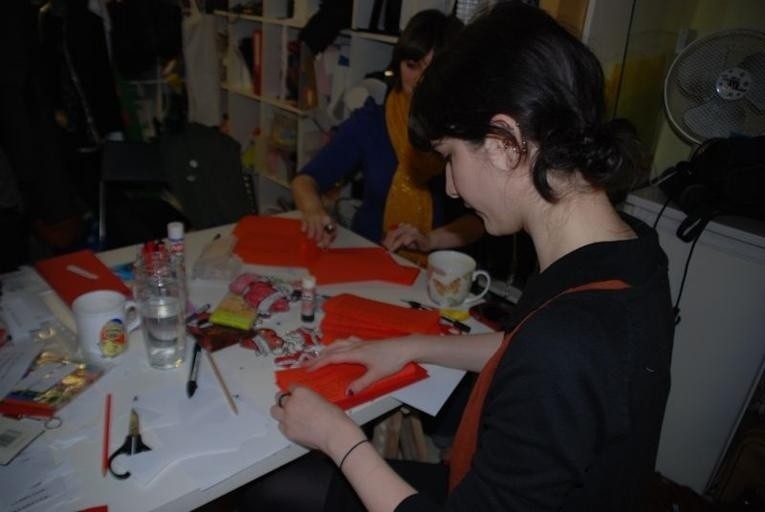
[{"x1": 338, "y1": 438, "x2": 370, "y2": 472}]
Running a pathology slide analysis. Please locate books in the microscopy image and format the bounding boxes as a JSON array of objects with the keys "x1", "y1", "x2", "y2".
[
  {"x1": 249, "y1": 29, "x2": 263, "y2": 98},
  {"x1": 368, "y1": 0, "x2": 401, "y2": 34}
]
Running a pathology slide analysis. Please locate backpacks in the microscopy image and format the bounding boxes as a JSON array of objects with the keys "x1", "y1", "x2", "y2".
[{"x1": 654, "y1": 135, "x2": 765, "y2": 241}]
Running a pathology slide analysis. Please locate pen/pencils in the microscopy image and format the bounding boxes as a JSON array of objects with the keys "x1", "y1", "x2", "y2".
[
  {"x1": 184, "y1": 303, "x2": 210, "y2": 324},
  {"x1": 187, "y1": 342, "x2": 201, "y2": 398},
  {"x1": 101, "y1": 394, "x2": 111, "y2": 477},
  {"x1": 135, "y1": 238, "x2": 178, "y2": 297},
  {"x1": 206, "y1": 353, "x2": 239, "y2": 416},
  {"x1": 199, "y1": 233, "x2": 221, "y2": 257},
  {"x1": 409, "y1": 301, "x2": 470, "y2": 333}
]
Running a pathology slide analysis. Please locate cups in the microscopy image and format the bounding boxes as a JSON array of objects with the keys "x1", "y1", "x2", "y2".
[
  {"x1": 131, "y1": 251, "x2": 189, "y2": 371},
  {"x1": 425, "y1": 250, "x2": 491, "y2": 309},
  {"x1": 71, "y1": 289, "x2": 142, "y2": 364}
]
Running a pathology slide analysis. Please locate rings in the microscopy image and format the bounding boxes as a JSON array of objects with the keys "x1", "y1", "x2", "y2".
[
  {"x1": 276, "y1": 391, "x2": 291, "y2": 406},
  {"x1": 324, "y1": 223, "x2": 335, "y2": 236}
]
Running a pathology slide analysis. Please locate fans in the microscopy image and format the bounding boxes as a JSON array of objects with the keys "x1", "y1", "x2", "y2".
[{"x1": 664, "y1": 28, "x2": 765, "y2": 149}]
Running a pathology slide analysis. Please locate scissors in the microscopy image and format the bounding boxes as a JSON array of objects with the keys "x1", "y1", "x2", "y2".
[
  {"x1": 107, "y1": 395, "x2": 151, "y2": 478},
  {"x1": 401, "y1": 300, "x2": 470, "y2": 321}
]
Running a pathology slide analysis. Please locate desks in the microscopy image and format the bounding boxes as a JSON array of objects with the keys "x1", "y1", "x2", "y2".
[{"x1": 0, "y1": 209, "x2": 502, "y2": 512}]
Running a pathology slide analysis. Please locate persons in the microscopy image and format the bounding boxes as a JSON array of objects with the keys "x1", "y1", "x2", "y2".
[
  {"x1": 288, "y1": 8, "x2": 508, "y2": 272},
  {"x1": 271, "y1": 0, "x2": 674, "y2": 510}
]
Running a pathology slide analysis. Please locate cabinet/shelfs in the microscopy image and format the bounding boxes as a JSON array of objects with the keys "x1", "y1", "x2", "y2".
[
  {"x1": 176, "y1": 1, "x2": 635, "y2": 216},
  {"x1": 622, "y1": 202, "x2": 764, "y2": 499}
]
